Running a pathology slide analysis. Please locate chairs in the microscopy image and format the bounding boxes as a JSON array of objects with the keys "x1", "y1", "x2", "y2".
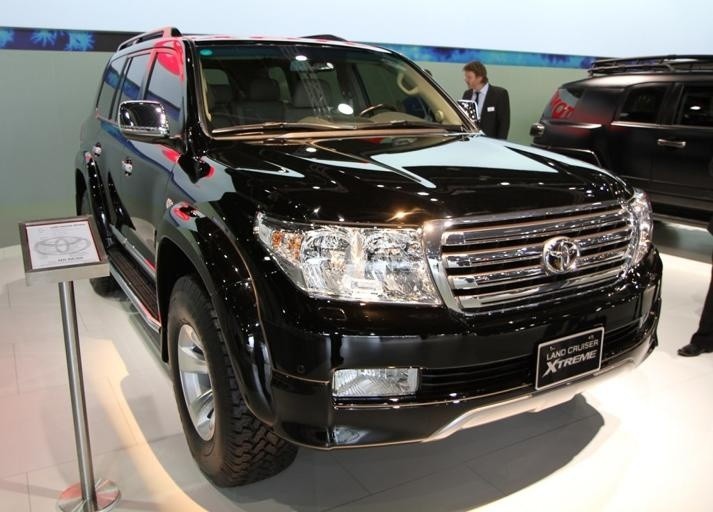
[{"x1": 207, "y1": 78, "x2": 342, "y2": 127}]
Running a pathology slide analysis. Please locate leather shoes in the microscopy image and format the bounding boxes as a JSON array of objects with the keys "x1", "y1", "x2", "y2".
[{"x1": 678, "y1": 343, "x2": 712, "y2": 357}]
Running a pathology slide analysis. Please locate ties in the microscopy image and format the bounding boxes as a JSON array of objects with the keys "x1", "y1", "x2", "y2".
[{"x1": 475, "y1": 92, "x2": 478, "y2": 105}]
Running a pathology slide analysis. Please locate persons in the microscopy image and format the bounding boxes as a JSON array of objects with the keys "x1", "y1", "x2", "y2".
[
  {"x1": 674, "y1": 211, "x2": 712, "y2": 359},
  {"x1": 399, "y1": 68, "x2": 436, "y2": 120},
  {"x1": 460, "y1": 62, "x2": 512, "y2": 143}
]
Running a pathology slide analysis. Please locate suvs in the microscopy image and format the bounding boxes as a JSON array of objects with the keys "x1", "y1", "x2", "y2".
[
  {"x1": 527, "y1": 52, "x2": 713, "y2": 238},
  {"x1": 71, "y1": 28, "x2": 664, "y2": 491}
]
